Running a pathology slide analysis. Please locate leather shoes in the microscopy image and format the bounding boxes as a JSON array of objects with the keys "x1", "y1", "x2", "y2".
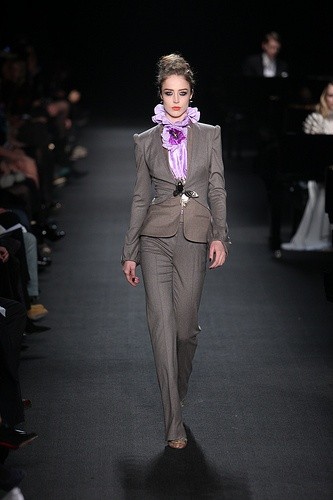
[{"x1": 36, "y1": 202, "x2": 66, "y2": 271}]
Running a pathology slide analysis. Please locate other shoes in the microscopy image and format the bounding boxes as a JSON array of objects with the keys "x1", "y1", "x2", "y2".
[
  {"x1": 22, "y1": 398, "x2": 33, "y2": 409},
  {"x1": 27, "y1": 304, "x2": 48, "y2": 319}
]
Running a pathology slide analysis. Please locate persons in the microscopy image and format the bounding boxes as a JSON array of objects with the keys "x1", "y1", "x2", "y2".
[
  {"x1": 0, "y1": 43, "x2": 89, "y2": 500},
  {"x1": 118, "y1": 51, "x2": 235, "y2": 450},
  {"x1": 248, "y1": 27, "x2": 333, "y2": 302}
]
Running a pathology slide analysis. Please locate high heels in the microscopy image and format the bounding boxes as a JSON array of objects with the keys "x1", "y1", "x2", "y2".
[
  {"x1": 0, "y1": 423, "x2": 38, "y2": 449},
  {"x1": 0, "y1": 465, "x2": 26, "y2": 492},
  {"x1": 168, "y1": 437, "x2": 187, "y2": 453}
]
